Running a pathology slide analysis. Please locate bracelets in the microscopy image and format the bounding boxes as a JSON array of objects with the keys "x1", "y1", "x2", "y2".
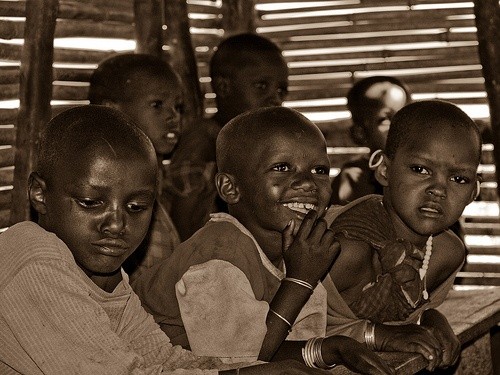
[
  {"x1": 364, "y1": 322, "x2": 376, "y2": 352},
  {"x1": 301, "y1": 336, "x2": 337, "y2": 370},
  {"x1": 269, "y1": 308, "x2": 293, "y2": 332},
  {"x1": 281, "y1": 277, "x2": 315, "y2": 291}
]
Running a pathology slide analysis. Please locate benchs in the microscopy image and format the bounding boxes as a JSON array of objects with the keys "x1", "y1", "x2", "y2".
[{"x1": 327, "y1": 286, "x2": 500, "y2": 375}]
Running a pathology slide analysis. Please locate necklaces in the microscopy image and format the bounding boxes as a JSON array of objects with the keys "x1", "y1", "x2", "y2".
[{"x1": 412, "y1": 233, "x2": 433, "y2": 300}]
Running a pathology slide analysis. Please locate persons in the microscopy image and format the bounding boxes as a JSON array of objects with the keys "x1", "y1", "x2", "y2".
[
  {"x1": 84, "y1": 51, "x2": 182, "y2": 284},
  {"x1": 1, "y1": 106, "x2": 331, "y2": 375},
  {"x1": 326, "y1": 99, "x2": 481, "y2": 371},
  {"x1": 130, "y1": 107, "x2": 396, "y2": 375},
  {"x1": 326, "y1": 76, "x2": 412, "y2": 207},
  {"x1": 175, "y1": 33, "x2": 290, "y2": 238}
]
